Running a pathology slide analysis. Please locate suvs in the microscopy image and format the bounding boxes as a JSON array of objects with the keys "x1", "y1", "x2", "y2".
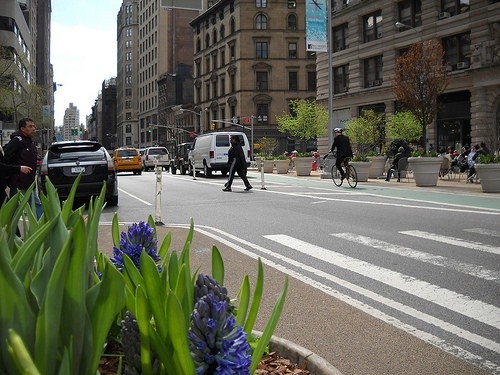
[
  {"x1": 112, "y1": 147, "x2": 142, "y2": 175},
  {"x1": 141, "y1": 147, "x2": 170, "y2": 171},
  {"x1": 170, "y1": 142, "x2": 194, "y2": 175}
]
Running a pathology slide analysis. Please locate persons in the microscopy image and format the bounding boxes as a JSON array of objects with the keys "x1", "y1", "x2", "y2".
[
  {"x1": 222, "y1": 135, "x2": 253, "y2": 192},
  {"x1": 429, "y1": 144, "x2": 434, "y2": 151},
  {"x1": 4, "y1": 117, "x2": 44, "y2": 220},
  {"x1": 418, "y1": 145, "x2": 423, "y2": 150},
  {"x1": 385, "y1": 147, "x2": 408, "y2": 182},
  {"x1": 437, "y1": 142, "x2": 494, "y2": 183},
  {"x1": 329, "y1": 128, "x2": 353, "y2": 180},
  {"x1": 0, "y1": 146, "x2": 33, "y2": 240}
]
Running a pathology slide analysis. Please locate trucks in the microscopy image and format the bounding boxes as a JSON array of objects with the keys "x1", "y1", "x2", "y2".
[{"x1": 187, "y1": 131, "x2": 251, "y2": 177}]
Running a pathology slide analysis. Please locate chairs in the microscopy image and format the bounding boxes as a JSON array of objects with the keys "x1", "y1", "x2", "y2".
[{"x1": 438, "y1": 157, "x2": 451, "y2": 181}]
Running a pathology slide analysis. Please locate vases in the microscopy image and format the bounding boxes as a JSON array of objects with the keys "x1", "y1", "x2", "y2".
[{"x1": 250, "y1": 330, "x2": 344, "y2": 375}]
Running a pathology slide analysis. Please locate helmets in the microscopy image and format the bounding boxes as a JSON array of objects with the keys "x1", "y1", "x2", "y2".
[{"x1": 333, "y1": 128, "x2": 342, "y2": 133}]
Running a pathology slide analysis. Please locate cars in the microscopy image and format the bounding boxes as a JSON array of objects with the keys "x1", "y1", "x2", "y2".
[{"x1": 39, "y1": 138, "x2": 120, "y2": 209}]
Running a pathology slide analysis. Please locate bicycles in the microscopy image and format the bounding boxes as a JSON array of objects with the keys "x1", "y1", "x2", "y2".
[{"x1": 328, "y1": 150, "x2": 357, "y2": 188}]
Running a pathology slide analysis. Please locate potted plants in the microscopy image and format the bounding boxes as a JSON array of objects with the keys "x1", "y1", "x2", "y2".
[
  {"x1": 474, "y1": 152, "x2": 500, "y2": 193},
  {"x1": 344, "y1": 110, "x2": 424, "y2": 182},
  {"x1": 407, "y1": 149, "x2": 443, "y2": 187},
  {"x1": 254, "y1": 98, "x2": 330, "y2": 177}
]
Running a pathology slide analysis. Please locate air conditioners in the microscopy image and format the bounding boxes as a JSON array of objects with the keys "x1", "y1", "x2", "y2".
[
  {"x1": 438, "y1": 12, "x2": 451, "y2": 19},
  {"x1": 368, "y1": 80, "x2": 381, "y2": 87},
  {"x1": 441, "y1": 61, "x2": 469, "y2": 73},
  {"x1": 377, "y1": 31, "x2": 383, "y2": 38}
]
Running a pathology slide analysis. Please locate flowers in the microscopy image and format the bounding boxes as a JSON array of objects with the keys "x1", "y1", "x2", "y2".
[{"x1": 0, "y1": 169, "x2": 289, "y2": 375}]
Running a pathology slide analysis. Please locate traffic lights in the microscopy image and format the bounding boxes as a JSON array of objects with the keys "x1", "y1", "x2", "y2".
[
  {"x1": 148, "y1": 125, "x2": 152, "y2": 132},
  {"x1": 171, "y1": 105, "x2": 183, "y2": 115},
  {"x1": 210, "y1": 123, "x2": 215, "y2": 130}
]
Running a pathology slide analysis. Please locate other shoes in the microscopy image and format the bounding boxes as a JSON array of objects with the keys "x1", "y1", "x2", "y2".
[
  {"x1": 397, "y1": 180, "x2": 400, "y2": 182},
  {"x1": 222, "y1": 187, "x2": 231, "y2": 191},
  {"x1": 384, "y1": 179, "x2": 388, "y2": 181},
  {"x1": 244, "y1": 185, "x2": 252, "y2": 191},
  {"x1": 341, "y1": 174, "x2": 346, "y2": 181}
]
